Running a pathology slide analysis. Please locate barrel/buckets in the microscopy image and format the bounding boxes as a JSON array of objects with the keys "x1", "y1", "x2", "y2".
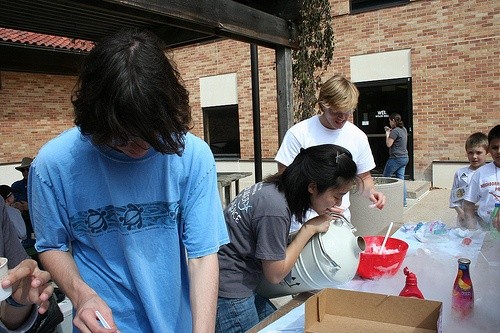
[{"x1": 252, "y1": 212, "x2": 366, "y2": 299}]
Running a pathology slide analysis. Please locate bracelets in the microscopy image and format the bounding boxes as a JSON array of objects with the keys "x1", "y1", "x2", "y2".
[{"x1": 5, "y1": 296, "x2": 28, "y2": 307}]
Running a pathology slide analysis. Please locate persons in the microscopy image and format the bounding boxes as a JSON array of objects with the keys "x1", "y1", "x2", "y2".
[
  {"x1": 0, "y1": 158, "x2": 65, "y2": 333},
  {"x1": 463, "y1": 125, "x2": 500, "y2": 233},
  {"x1": 383, "y1": 113, "x2": 409, "y2": 207},
  {"x1": 212, "y1": 144, "x2": 364, "y2": 333},
  {"x1": 274, "y1": 75, "x2": 386, "y2": 236},
  {"x1": 27, "y1": 26, "x2": 231, "y2": 333},
  {"x1": 449, "y1": 132, "x2": 490, "y2": 231}
]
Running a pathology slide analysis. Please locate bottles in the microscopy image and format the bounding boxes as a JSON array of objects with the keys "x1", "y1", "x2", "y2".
[
  {"x1": 489, "y1": 203, "x2": 500, "y2": 238},
  {"x1": 452, "y1": 258, "x2": 474, "y2": 319},
  {"x1": 398, "y1": 266, "x2": 424, "y2": 300}
]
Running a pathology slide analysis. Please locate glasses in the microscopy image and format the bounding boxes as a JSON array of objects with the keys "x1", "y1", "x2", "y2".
[
  {"x1": 21, "y1": 168, "x2": 29, "y2": 172},
  {"x1": 112, "y1": 134, "x2": 146, "y2": 145}
]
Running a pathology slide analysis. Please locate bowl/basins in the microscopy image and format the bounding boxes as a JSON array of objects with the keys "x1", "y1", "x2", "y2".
[{"x1": 356, "y1": 235, "x2": 409, "y2": 281}]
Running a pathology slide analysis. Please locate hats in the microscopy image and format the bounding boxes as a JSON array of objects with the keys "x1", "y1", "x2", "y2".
[
  {"x1": 0, "y1": 184, "x2": 15, "y2": 199},
  {"x1": 15, "y1": 156, "x2": 33, "y2": 170}
]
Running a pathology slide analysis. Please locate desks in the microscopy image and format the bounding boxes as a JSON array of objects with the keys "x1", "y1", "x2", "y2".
[
  {"x1": 217, "y1": 172, "x2": 252, "y2": 206},
  {"x1": 244, "y1": 220, "x2": 500, "y2": 333}
]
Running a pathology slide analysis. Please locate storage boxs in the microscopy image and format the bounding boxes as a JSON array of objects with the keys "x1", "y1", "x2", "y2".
[{"x1": 304, "y1": 288, "x2": 443, "y2": 333}]
